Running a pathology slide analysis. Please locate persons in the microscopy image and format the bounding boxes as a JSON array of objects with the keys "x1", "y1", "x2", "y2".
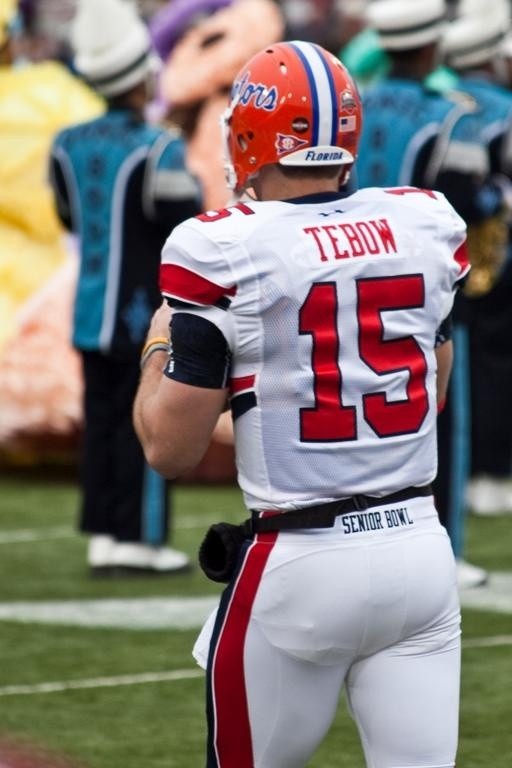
[
  {"x1": 132, "y1": 40, "x2": 470, "y2": 768},
  {"x1": 47, "y1": 0, "x2": 511, "y2": 590}
]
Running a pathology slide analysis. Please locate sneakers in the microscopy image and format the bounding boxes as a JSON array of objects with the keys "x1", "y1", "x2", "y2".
[{"x1": 87, "y1": 535, "x2": 192, "y2": 578}]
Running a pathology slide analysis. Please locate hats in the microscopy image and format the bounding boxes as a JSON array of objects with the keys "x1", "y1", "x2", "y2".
[
  {"x1": 71, "y1": 18, "x2": 161, "y2": 99},
  {"x1": 368, "y1": 0, "x2": 511, "y2": 73}
]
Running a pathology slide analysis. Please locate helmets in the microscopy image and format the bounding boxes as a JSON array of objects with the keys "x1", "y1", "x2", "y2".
[{"x1": 220, "y1": 40, "x2": 363, "y2": 205}]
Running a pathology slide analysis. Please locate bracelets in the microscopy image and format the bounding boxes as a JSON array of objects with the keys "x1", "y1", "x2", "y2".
[{"x1": 139, "y1": 337, "x2": 175, "y2": 363}]
